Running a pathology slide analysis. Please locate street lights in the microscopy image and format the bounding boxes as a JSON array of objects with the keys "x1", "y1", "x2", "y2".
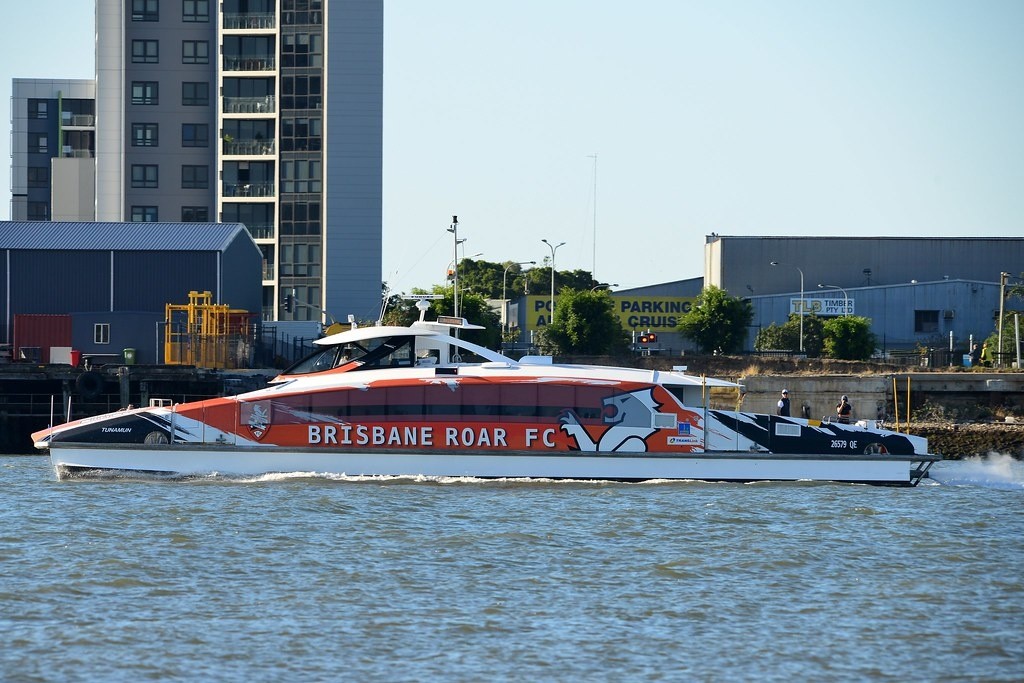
[
  {"x1": 542, "y1": 239, "x2": 570, "y2": 325},
  {"x1": 501, "y1": 260, "x2": 537, "y2": 340},
  {"x1": 447, "y1": 250, "x2": 485, "y2": 354},
  {"x1": 819, "y1": 283, "x2": 849, "y2": 318},
  {"x1": 770, "y1": 261, "x2": 804, "y2": 351}
]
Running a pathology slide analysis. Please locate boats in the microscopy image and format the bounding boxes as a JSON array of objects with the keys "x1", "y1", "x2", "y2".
[{"x1": 26, "y1": 318, "x2": 946, "y2": 489}]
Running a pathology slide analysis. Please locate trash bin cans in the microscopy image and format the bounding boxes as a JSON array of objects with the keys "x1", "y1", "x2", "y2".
[{"x1": 123, "y1": 348, "x2": 136, "y2": 365}]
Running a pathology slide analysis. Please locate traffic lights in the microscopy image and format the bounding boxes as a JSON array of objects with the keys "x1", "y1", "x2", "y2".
[
  {"x1": 636, "y1": 335, "x2": 648, "y2": 344},
  {"x1": 643, "y1": 333, "x2": 655, "y2": 342}
]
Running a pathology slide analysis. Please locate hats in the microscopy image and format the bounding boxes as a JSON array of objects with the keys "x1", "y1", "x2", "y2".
[{"x1": 782, "y1": 389, "x2": 788, "y2": 394}]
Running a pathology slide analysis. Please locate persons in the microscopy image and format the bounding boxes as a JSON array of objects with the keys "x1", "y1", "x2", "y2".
[
  {"x1": 969, "y1": 344, "x2": 981, "y2": 366},
  {"x1": 339, "y1": 355, "x2": 349, "y2": 364},
  {"x1": 121, "y1": 403, "x2": 134, "y2": 411},
  {"x1": 980, "y1": 342, "x2": 992, "y2": 367},
  {"x1": 389, "y1": 354, "x2": 399, "y2": 364},
  {"x1": 777, "y1": 389, "x2": 790, "y2": 416},
  {"x1": 836, "y1": 395, "x2": 851, "y2": 424}
]
